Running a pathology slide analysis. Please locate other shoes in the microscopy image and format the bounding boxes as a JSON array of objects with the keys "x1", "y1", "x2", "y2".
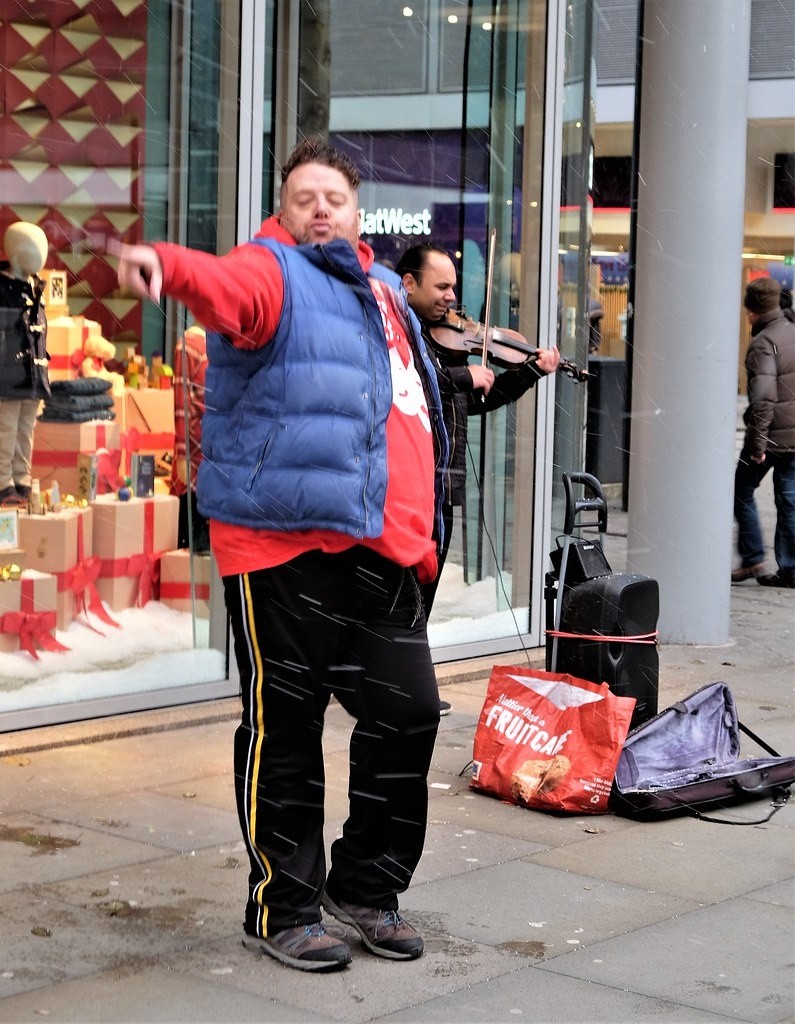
[
  {"x1": 0, "y1": 484, "x2": 31, "y2": 505},
  {"x1": 757, "y1": 569, "x2": 795, "y2": 588},
  {"x1": 731, "y1": 563, "x2": 765, "y2": 582}
]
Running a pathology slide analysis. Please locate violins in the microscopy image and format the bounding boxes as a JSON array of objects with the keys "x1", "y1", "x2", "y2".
[{"x1": 429, "y1": 306, "x2": 598, "y2": 384}]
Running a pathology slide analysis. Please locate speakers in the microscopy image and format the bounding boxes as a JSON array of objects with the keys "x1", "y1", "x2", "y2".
[
  {"x1": 550, "y1": 539, "x2": 611, "y2": 587},
  {"x1": 557, "y1": 574, "x2": 660, "y2": 733}
]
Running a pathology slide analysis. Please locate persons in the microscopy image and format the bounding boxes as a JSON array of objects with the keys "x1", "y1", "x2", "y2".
[
  {"x1": 735, "y1": 276, "x2": 795, "y2": 587},
  {"x1": 105, "y1": 143, "x2": 452, "y2": 972},
  {"x1": 0, "y1": 222, "x2": 48, "y2": 507},
  {"x1": 169, "y1": 318, "x2": 208, "y2": 554},
  {"x1": 399, "y1": 246, "x2": 561, "y2": 717}
]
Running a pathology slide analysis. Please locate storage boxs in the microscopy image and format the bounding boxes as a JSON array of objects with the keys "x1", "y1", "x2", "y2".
[
  {"x1": 160, "y1": 548, "x2": 210, "y2": 619},
  {"x1": 0, "y1": 569, "x2": 57, "y2": 653},
  {"x1": 31, "y1": 419, "x2": 119, "y2": 501},
  {"x1": 87, "y1": 492, "x2": 180, "y2": 613},
  {"x1": 126, "y1": 387, "x2": 174, "y2": 481},
  {"x1": 46, "y1": 316, "x2": 101, "y2": 386},
  {"x1": 0, "y1": 506, "x2": 94, "y2": 632}
]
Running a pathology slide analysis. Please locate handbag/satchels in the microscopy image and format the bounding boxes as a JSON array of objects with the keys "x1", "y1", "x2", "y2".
[{"x1": 468, "y1": 666, "x2": 636, "y2": 813}]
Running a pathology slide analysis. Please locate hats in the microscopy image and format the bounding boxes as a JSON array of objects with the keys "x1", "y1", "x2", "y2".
[{"x1": 744, "y1": 278, "x2": 780, "y2": 315}]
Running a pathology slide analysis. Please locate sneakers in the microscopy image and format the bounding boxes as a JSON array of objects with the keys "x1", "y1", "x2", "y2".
[
  {"x1": 242, "y1": 924, "x2": 353, "y2": 970},
  {"x1": 322, "y1": 887, "x2": 424, "y2": 958}
]
